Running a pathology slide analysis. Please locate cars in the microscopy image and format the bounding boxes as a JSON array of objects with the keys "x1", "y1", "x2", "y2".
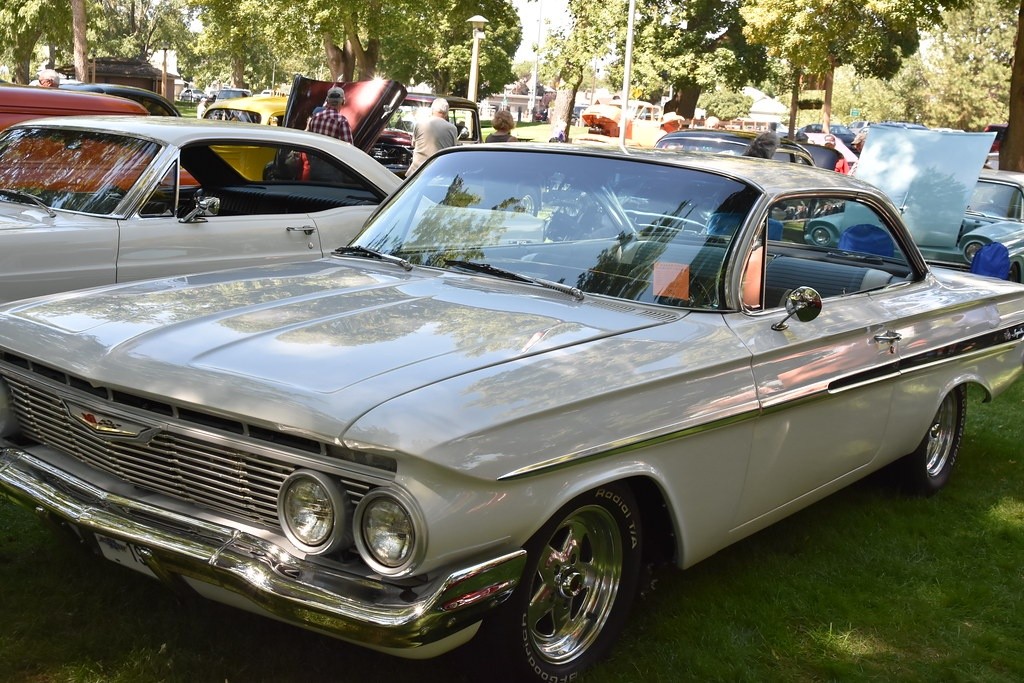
[{"x1": 0, "y1": 81, "x2": 1024, "y2": 682}]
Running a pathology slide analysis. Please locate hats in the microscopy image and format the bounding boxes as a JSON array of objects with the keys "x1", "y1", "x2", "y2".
[
  {"x1": 326, "y1": 87, "x2": 344, "y2": 102},
  {"x1": 661, "y1": 112, "x2": 685, "y2": 125},
  {"x1": 851, "y1": 132, "x2": 866, "y2": 146}
]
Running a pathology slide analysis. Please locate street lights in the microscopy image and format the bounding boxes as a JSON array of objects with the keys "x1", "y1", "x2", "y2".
[{"x1": 463, "y1": 15, "x2": 490, "y2": 138}]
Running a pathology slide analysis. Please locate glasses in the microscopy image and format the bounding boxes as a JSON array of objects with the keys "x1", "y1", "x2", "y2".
[
  {"x1": 39, "y1": 79, "x2": 46, "y2": 84},
  {"x1": 669, "y1": 123, "x2": 680, "y2": 128}
]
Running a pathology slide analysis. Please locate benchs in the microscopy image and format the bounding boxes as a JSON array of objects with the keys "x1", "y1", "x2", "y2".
[
  {"x1": 622, "y1": 241, "x2": 891, "y2": 311},
  {"x1": 192, "y1": 187, "x2": 372, "y2": 217}
]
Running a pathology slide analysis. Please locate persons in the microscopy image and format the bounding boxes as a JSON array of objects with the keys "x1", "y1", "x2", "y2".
[
  {"x1": 197, "y1": 98, "x2": 207, "y2": 119},
  {"x1": 485, "y1": 110, "x2": 518, "y2": 143},
  {"x1": 38, "y1": 69, "x2": 60, "y2": 87},
  {"x1": 795, "y1": 131, "x2": 810, "y2": 144},
  {"x1": 403, "y1": 98, "x2": 458, "y2": 177},
  {"x1": 300, "y1": 87, "x2": 353, "y2": 183},
  {"x1": 824, "y1": 134, "x2": 836, "y2": 148}
]
